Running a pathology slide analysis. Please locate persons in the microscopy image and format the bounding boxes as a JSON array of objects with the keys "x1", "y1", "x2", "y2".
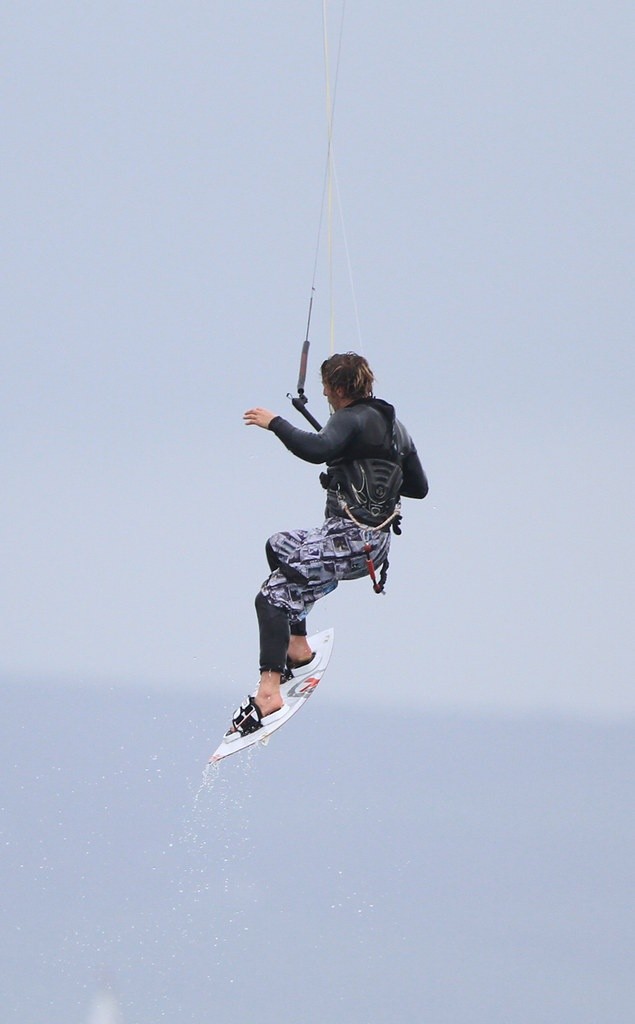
[{"x1": 230, "y1": 351, "x2": 428, "y2": 730}]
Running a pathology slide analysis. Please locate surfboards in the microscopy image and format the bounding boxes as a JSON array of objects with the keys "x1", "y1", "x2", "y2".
[{"x1": 207, "y1": 625, "x2": 336, "y2": 767}]
